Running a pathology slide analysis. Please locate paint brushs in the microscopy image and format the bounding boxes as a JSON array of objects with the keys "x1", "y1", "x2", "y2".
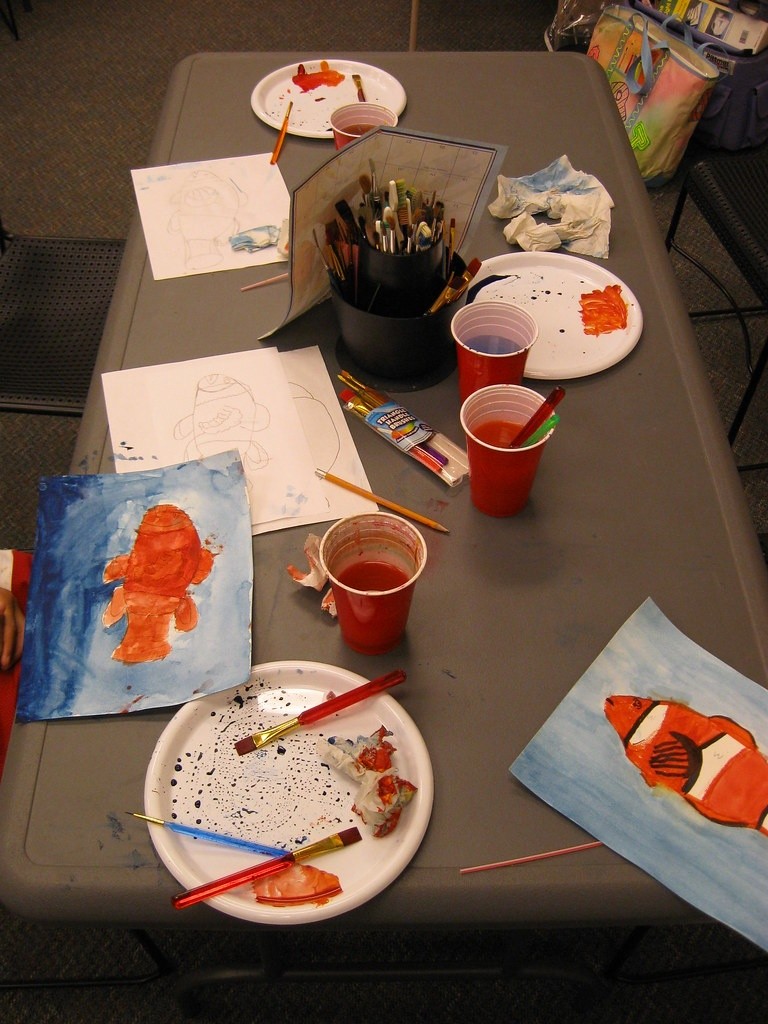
[
  {"x1": 338, "y1": 369, "x2": 458, "y2": 486},
  {"x1": 125, "y1": 809, "x2": 288, "y2": 857},
  {"x1": 172, "y1": 827, "x2": 362, "y2": 909},
  {"x1": 311, "y1": 156, "x2": 482, "y2": 314},
  {"x1": 233, "y1": 669, "x2": 407, "y2": 755},
  {"x1": 352, "y1": 74, "x2": 366, "y2": 104},
  {"x1": 270, "y1": 100, "x2": 295, "y2": 166}
]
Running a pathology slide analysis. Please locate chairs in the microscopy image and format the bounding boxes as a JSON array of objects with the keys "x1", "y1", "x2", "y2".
[
  {"x1": 666, "y1": 140, "x2": 768, "y2": 474},
  {"x1": 1, "y1": 222, "x2": 126, "y2": 416}
]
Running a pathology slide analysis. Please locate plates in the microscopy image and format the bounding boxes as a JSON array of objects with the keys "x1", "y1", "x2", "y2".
[
  {"x1": 251, "y1": 59, "x2": 406, "y2": 138},
  {"x1": 469, "y1": 252, "x2": 643, "y2": 379},
  {"x1": 144, "y1": 661, "x2": 433, "y2": 924}
]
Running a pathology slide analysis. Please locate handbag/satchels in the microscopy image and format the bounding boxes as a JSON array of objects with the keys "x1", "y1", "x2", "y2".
[
  {"x1": 629, "y1": 0, "x2": 768, "y2": 149},
  {"x1": 587, "y1": 4, "x2": 730, "y2": 187}
]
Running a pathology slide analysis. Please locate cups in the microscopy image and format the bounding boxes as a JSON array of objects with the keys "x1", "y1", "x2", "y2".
[
  {"x1": 319, "y1": 512, "x2": 427, "y2": 655},
  {"x1": 330, "y1": 102, "x2": 398, "y2": 150},
  {"x1": 451, "y1": 301, "x2": 539, "y2": 405},
  {"x1": 354, "y1": 231, "x2": 441, "y2": 296},
  {"x1": 460, "y1": 384, "x2": 556, "y2": 517}
]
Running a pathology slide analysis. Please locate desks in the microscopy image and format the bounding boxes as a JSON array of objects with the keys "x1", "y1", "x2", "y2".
[{"x1": 0, "y1": 53, "x2": 768, "y2": 1024}]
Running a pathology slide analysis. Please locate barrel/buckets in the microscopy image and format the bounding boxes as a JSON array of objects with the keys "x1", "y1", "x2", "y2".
[{"x1": 329, "y1": 248, "x2": 471, "y2": 392}]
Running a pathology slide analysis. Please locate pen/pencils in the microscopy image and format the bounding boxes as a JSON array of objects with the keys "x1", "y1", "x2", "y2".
[{"x1": 315, "y1": 469, "x2": 449, "y2": 532}]
